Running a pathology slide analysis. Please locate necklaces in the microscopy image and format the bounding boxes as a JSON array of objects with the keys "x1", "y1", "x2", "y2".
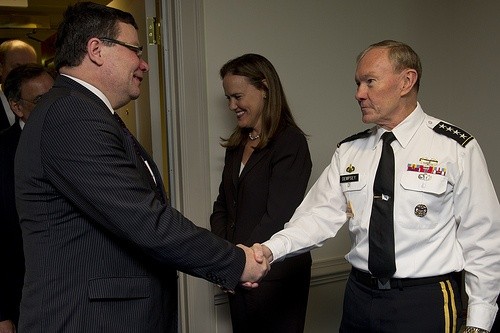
[{"x1": 249, "y1": 133, "x2": 260, "y2": 140}]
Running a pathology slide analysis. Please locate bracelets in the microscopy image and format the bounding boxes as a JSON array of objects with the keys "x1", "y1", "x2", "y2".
[{"x1": 464, "y1": 326, "x2": 489, "y2": 333}]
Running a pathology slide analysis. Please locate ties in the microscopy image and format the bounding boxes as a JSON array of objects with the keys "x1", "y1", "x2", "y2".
[{"x1": 368, "y1": 132, "x2": 396, "y2": 286}]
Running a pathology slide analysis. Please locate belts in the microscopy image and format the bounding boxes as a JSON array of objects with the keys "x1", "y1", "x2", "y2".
[{"x1": 350, "y1": 268, "x2": 455, "y2": 287}]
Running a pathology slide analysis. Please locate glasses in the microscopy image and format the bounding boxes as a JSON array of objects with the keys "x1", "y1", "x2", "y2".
[
  {"x1": 84, "y1": 37, "x2": 143, "y2": 59},
  {"x1": 13, "y1": 96, "x2": 42, "y2": 105}
]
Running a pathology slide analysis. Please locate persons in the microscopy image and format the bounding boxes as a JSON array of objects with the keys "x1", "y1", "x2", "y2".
[
  {"x1": 0, "y1": 0, "x2": 271, "y2": 333},
  {"x1": 240, "y1": 41, "x2": 500, "y2": 333},
  {"x1": 210, "y1": 54, "x2": 312, "y2": 333}
]
